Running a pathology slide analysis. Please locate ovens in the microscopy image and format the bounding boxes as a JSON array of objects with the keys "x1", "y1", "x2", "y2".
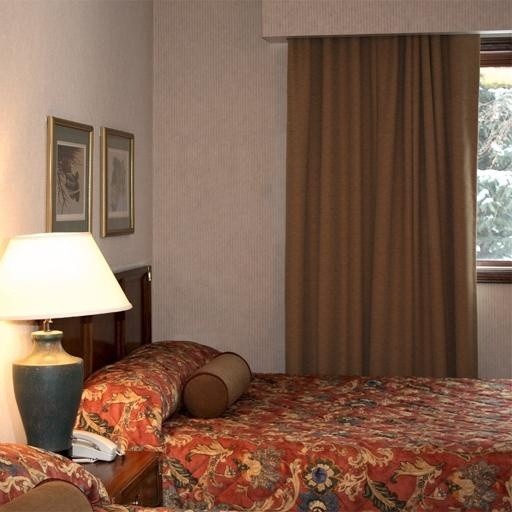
[{"x1": 38, "y1": 265, "x2": 512, "y2": 512}]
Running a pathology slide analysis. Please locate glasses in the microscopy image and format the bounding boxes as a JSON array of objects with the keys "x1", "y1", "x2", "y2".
[{"x1": 0, "y1": 232, "x2": 134, "y2": 453}]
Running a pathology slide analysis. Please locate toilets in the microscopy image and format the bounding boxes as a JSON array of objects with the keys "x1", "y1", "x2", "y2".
[{"x1": 71, "y1": 430, "x2": 126, "y2": 461}]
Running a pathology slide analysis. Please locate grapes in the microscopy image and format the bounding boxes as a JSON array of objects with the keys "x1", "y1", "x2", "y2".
[{"x1": 70, "y1": 451, "x2": 163, "y2": 507}]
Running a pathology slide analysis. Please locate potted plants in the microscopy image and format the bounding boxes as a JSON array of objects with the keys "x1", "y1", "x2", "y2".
[
  {"x1": 100, "y1": 126, "x2": 135, "y2": 238},
  {"x1": 45, "y1": 115, "x2": 94, "y2": 233}
]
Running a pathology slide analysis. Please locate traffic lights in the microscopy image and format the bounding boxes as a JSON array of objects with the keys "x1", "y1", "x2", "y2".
[
  {"x1": 0, "y1": 442, "x2": 112, "y2": 508},
  {"x1": 73, "y1": 340, "x2": 223, "y2": 451},
  {"x1": 182, "y1": 352, "x2": 253, "y2": 419},
  {"x1": 0, "y1": 479, "x2": 93, "y2": 512}
]
